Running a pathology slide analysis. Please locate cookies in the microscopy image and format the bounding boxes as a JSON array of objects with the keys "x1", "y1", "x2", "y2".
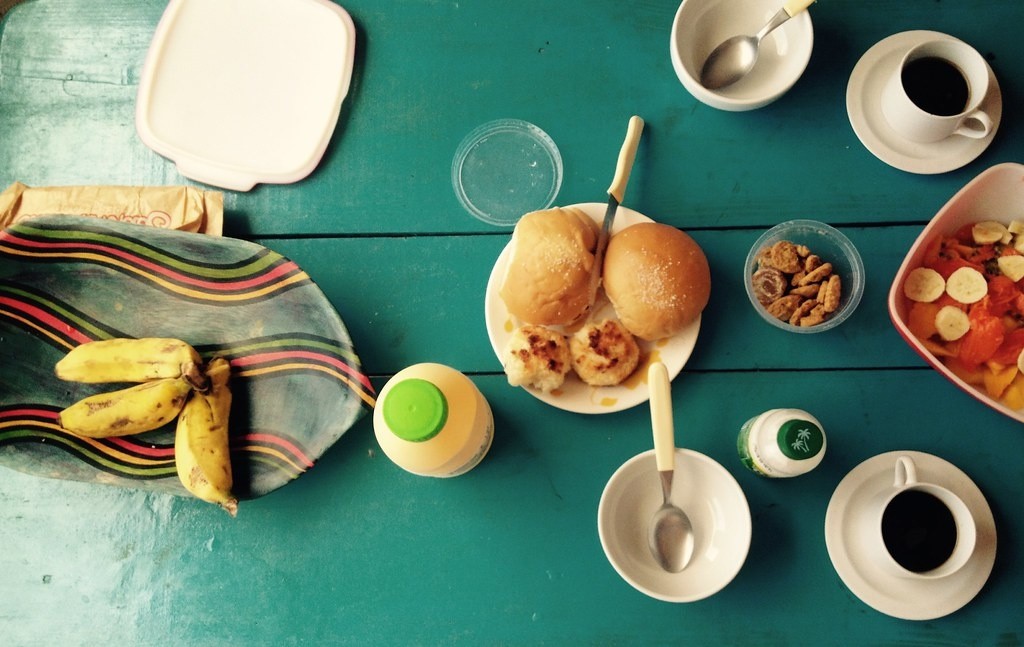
[{"x1": 751, "y1": 239, "x2": 842, "y2": 326}]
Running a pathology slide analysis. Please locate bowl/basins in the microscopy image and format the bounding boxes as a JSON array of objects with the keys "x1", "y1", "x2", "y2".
[
  {"x1": 669, "y1": 0, "x2": 814, "y2": 110},
  {"x1": 597, "y1": 446, "x2": 753, "y2": 603},
  {"x1": 888, "y1": 162, "x2": 1024, "y2": 423},
  {"x1": 743, "y1": 219, "x2": 865, "y2": 334}
]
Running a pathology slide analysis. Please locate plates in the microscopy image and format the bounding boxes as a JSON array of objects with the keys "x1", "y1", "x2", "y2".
[
  {"x1": 0, "y1": 211, "x2": 376, "y2": 502},
  {"x1": 825, "y1": 450, "x2": 997, "y2": 621},
  {"x1": 484, "y1": 202, "x2": 701, "y2": 414},
  {"x1": 845, "y1": 30, "x2": 1003, "y2": 174}
]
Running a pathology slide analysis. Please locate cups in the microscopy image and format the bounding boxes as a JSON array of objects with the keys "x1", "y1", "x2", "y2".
[
  {"x1": 860, "y1": 456, "x2": 977, "y2": 580},
  {"x1": 880, "y1": 38, "x2": 994, "y2": 143}
]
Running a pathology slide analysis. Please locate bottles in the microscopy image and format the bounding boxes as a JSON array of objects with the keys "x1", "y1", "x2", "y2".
[
  {"x1": 738, "y1": 408, "x2": 827, "y2": 479},
  {"x1": 373, "y1": 363, "x2": 494, "y2": 478}
]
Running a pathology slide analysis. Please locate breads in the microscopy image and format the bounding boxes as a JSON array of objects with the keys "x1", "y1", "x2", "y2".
[
  {"x1": 499, "y1": 205, "x2": 604, "y2": 326},
  {"x1": 603, "y1": 222, "x2": 712, "y2": 340},
  {"x1": 567, "y1": 317, "x2": 640, "y2": 386},
  {"x1": 504, "y1": 323, "x2": 571, "y2": 394}
]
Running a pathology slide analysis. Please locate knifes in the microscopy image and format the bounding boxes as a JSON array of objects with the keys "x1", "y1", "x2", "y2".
[{"x1": 587, "y1": 115, "x2": 645, "y2": 311}]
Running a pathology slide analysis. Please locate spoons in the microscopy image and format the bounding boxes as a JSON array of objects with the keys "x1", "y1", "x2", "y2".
[
  {"x1": 647, "y1": 361, "x2": 694, "y2": 574},
  {"x1": 700, "y1": 0, "x2": 816, "y2": 89}
]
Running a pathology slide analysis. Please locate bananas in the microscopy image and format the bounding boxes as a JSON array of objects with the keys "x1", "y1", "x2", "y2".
[
  {"x1": 54, "y1": 337, "x2": 241, "y2": 521},
  {"x1": 904, "y1": 221, "x2": 1024, "y2": 379}
]
